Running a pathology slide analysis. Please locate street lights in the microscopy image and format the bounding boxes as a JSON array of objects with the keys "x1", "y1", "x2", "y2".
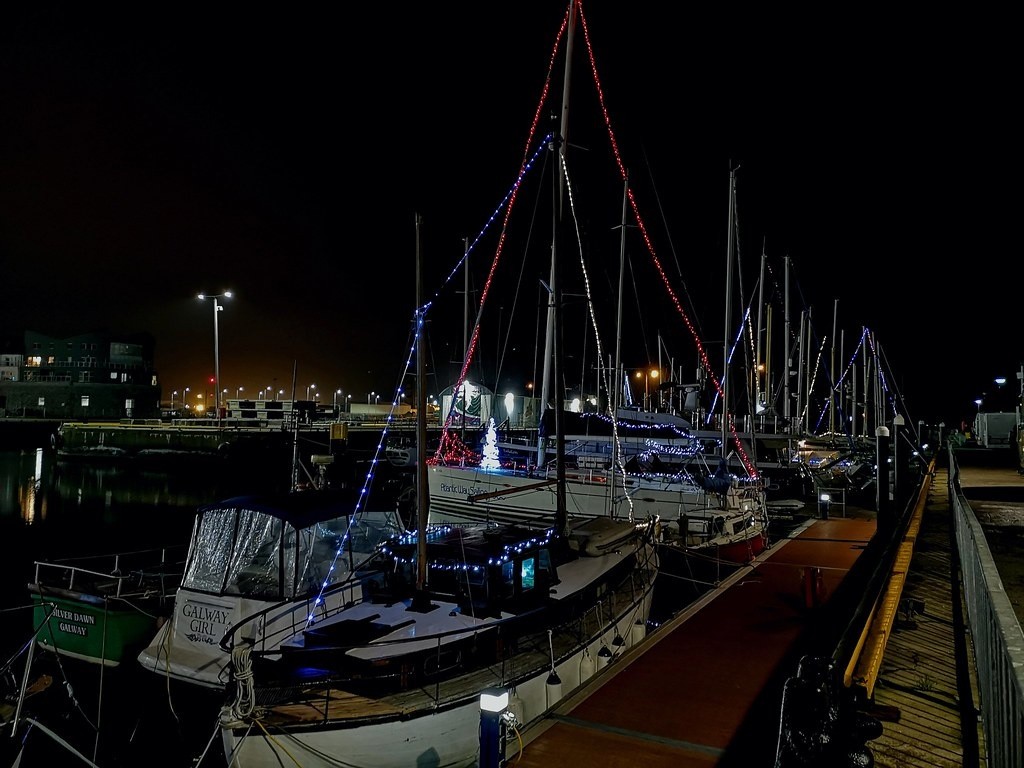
[{"x1": 975, "y1": 399, "x2": 982, "y2": 412}]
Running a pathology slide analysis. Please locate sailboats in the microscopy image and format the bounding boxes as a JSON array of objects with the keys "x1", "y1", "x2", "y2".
[{"x1": 0, "y1": 3, "x2": 940, "y2": 766}]
